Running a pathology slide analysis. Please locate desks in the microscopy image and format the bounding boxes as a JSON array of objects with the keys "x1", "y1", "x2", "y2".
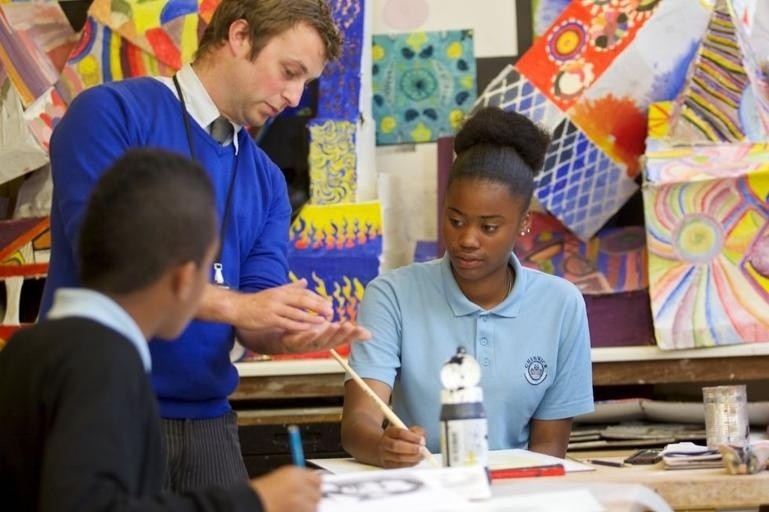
[
  {"x1": 313, "y1": 447, "x2": 769, "y2": 512},
  {"x1": 227, "y1": 342, "x2": 769, "y2": 425}
]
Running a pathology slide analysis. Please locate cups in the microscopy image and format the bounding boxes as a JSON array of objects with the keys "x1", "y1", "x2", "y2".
[{"x1": 702, "y1": 385, "x2": 749, "y2": 449}]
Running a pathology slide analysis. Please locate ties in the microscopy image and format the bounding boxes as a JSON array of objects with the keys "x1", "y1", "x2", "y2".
[{"x1": 210, "y1": 115, "x2": 234, "y2": 145}]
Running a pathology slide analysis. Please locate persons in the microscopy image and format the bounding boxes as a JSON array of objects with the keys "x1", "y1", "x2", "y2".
[
  {"x1": 1, "y1": 146, "x2": 322, "y2": 511},
  {"x1": 36, "y1": 0, "x2": 370, "y2": 486},
  {"x1": 339, "y1": 108, "x2": 593, "y2": 466}
]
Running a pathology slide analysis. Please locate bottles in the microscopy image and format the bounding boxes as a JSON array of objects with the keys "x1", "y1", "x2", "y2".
[{"x1": 441, "y1": 345, "x2": 488, "y2": 470}]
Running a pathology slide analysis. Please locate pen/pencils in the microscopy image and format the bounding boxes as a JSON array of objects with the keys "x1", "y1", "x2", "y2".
[
  {"x1": 587, "y1": 460, "x2": 632, "y2": 468},
  {"x1": 287, "y1": 424, "x2": 305, "y2": 468},
  {"x1": 330, "y1": 348, "x2": 436, "y2": 463}
]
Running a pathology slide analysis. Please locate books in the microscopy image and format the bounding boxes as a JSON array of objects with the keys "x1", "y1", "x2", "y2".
[{"x1": 659, "y1": 442, "x2": 724, "y2": 471}]
